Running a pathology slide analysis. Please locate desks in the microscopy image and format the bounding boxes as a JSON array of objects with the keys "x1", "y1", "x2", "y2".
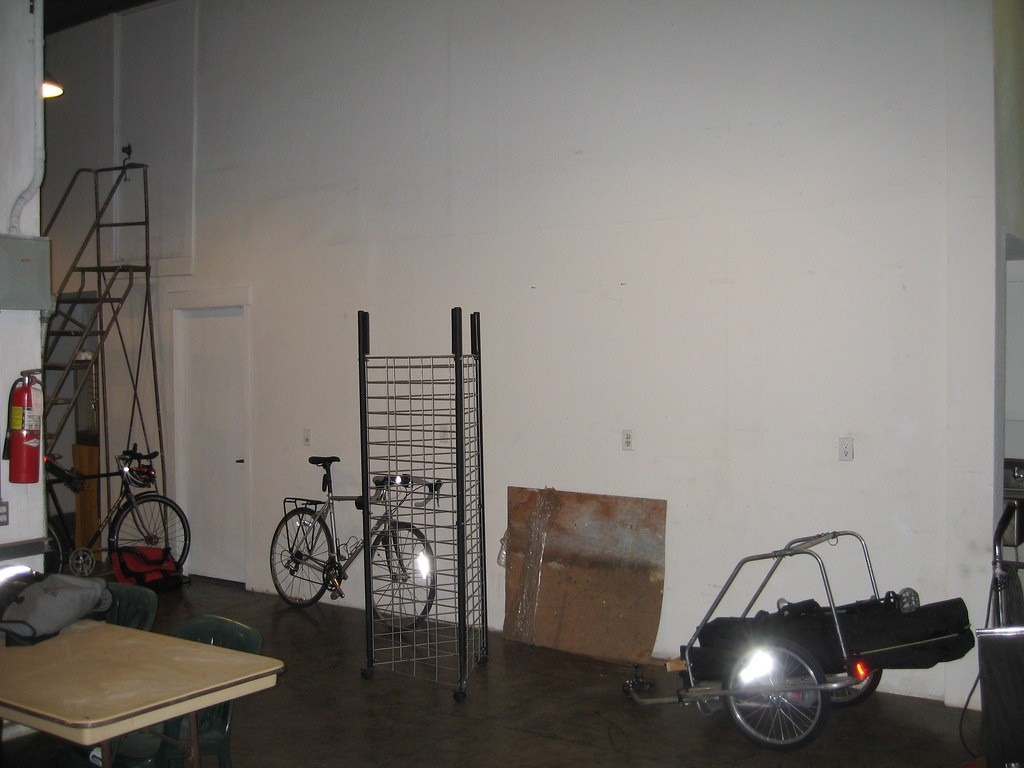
[{"x1": 0, "y1": 619, "x2": 284, "y2": 768}]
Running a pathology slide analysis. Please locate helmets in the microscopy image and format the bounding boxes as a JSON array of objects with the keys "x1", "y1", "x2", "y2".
[{"x1": 123, "y1": 466, "x2": 157, "y2": 489}]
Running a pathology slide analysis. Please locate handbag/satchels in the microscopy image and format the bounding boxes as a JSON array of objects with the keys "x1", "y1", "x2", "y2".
[
  {"x1": 111, "y1": 545, "x2": 183, "y2": 594},
  {"x1": 0, "y1": 571, "x2": 112, "y2": 646}
]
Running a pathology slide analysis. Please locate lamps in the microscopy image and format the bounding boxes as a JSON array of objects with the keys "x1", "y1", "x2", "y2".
[{"x1": 43, "y1": 71, "x2": 63, "y2": 98}]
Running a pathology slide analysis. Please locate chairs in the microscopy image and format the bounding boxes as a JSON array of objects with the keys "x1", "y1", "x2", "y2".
[
  {"x1": 91, "y1": 583, "x2": 158, "y2": 632},
  {"x1": 74, "y1": 614, "x2": 262, "y2": 767}
]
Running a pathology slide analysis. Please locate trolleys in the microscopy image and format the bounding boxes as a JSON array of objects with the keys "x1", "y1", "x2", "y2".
[{"x1": 621, "y1": 529, "x2": 889, "y2": 751}]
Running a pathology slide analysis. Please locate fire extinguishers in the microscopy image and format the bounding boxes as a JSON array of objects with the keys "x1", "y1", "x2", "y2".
[{"x1": 2, "y1": 368, "x2": 44, "y2": 484}]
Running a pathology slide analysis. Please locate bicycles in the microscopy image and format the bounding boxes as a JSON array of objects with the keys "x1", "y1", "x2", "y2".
[
  {"x1": 269, "y1": 454, "x2": 444, "y2": 635},
  {"x1": 39, "y1": 444, "x2": 190, "y2": 583}
]
